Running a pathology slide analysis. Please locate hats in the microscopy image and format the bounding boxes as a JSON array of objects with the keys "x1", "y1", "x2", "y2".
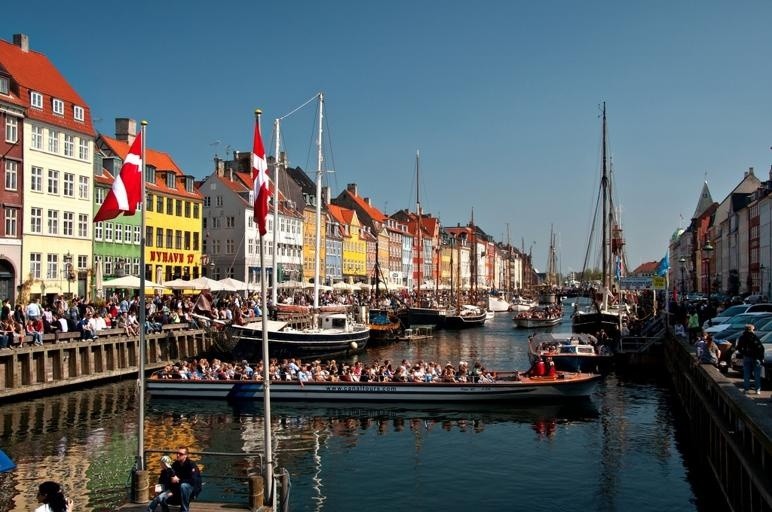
[{"x1": 157, "y1": 455, "x2": 171, "y2": 466}]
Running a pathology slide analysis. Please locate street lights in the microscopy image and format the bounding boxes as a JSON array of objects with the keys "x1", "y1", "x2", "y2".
[
  {"x1": 702, "y1": 238, "x2": 714, "y2": 327},
  {"x1": 118, "y1": 256, "x2": 126, "y2": 270},
  {"x1": 677, "y1": 256, "x2": 687, "y2": 321},
  {"x1": 65, "y1": 250, "x2": 72, "y2": 297}
]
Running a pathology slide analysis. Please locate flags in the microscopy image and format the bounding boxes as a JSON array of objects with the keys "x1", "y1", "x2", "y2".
[
  {"x1": 656, "y1": 252, "x2": 669, "y2": 278},
  {"x1": 202, "y1": 253, "x2": 210, "y2": 265},
  {"x1": 615, "y1": 254, "x2": 621, "y2": 281},
  {"x1": 249, "y1": 118, "x2": 270, "y2": 236},
  {"x1": 91, "y1": 132, "x2": 142, "y2": 223}
]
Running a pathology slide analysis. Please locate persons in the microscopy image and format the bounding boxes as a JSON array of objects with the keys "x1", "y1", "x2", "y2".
[
  {"x1": 534, "y1": 282, "x2": 767, "y2": 397},
  {"x1": 149, "y1": 456, "x2": 177, "y2": 511},
  {"x1": 31, "y1": 482, "x2": 73, "y2": 512},
  {"x1": 161, "y1": 447, "x2": 203, "y2": 511}
]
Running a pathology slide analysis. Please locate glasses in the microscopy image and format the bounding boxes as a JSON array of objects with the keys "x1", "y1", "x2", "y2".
[{"x1": 175, "y1": 452, "x2": 186, "y2": 456}]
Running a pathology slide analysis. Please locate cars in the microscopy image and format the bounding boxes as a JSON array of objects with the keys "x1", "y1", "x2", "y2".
[{"x1": 668, "y1": 291, "x2": 771, "y2": 373}]
